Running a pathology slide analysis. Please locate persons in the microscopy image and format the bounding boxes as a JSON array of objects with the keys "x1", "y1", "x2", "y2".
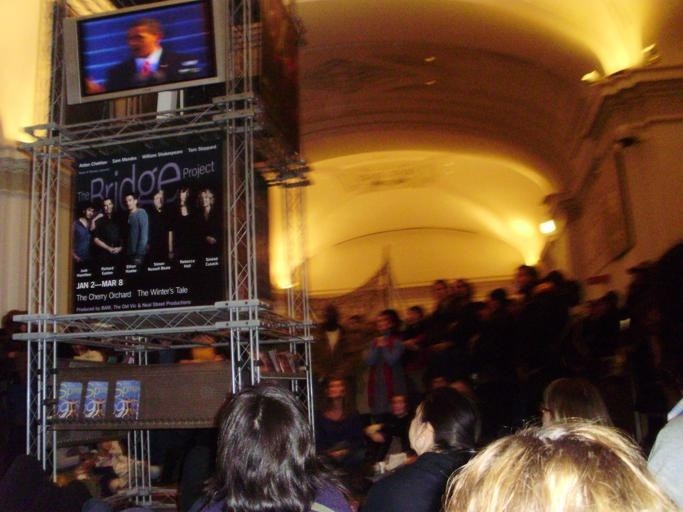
[
  {"x1": 81, "y1": 16, "x2": 190, "y2": 96},
  {"x1": 189, "y1": 382, "x2": 354, "y2": 512},
  {"x1": 313, "y1": 266, "x2": 663, "y2": 488},
  {"x1": 641, "y1": 235, "x2": 682, "y2": 509},
  {"x1": 444, "y1": 419, "x2": 683, "y2": 512},
  {"x1": 0, "y1": 310, "x2": 232, "y2": 512},
  {"x1": 66, "y1": 181, "x2": 219, "y2": 261},
  {"x1": 359, "y1": 386, "x2": 482, "y2": 512}
]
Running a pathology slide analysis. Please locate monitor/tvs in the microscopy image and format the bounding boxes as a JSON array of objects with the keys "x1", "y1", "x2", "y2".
[{"x1": 61, "y1": 0, "x2": 228, "y2": 105}]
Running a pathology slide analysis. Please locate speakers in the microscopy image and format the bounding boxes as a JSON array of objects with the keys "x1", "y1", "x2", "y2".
[{"x1": 55, "y1": 365, "x2": 231, "y2": 429}]
